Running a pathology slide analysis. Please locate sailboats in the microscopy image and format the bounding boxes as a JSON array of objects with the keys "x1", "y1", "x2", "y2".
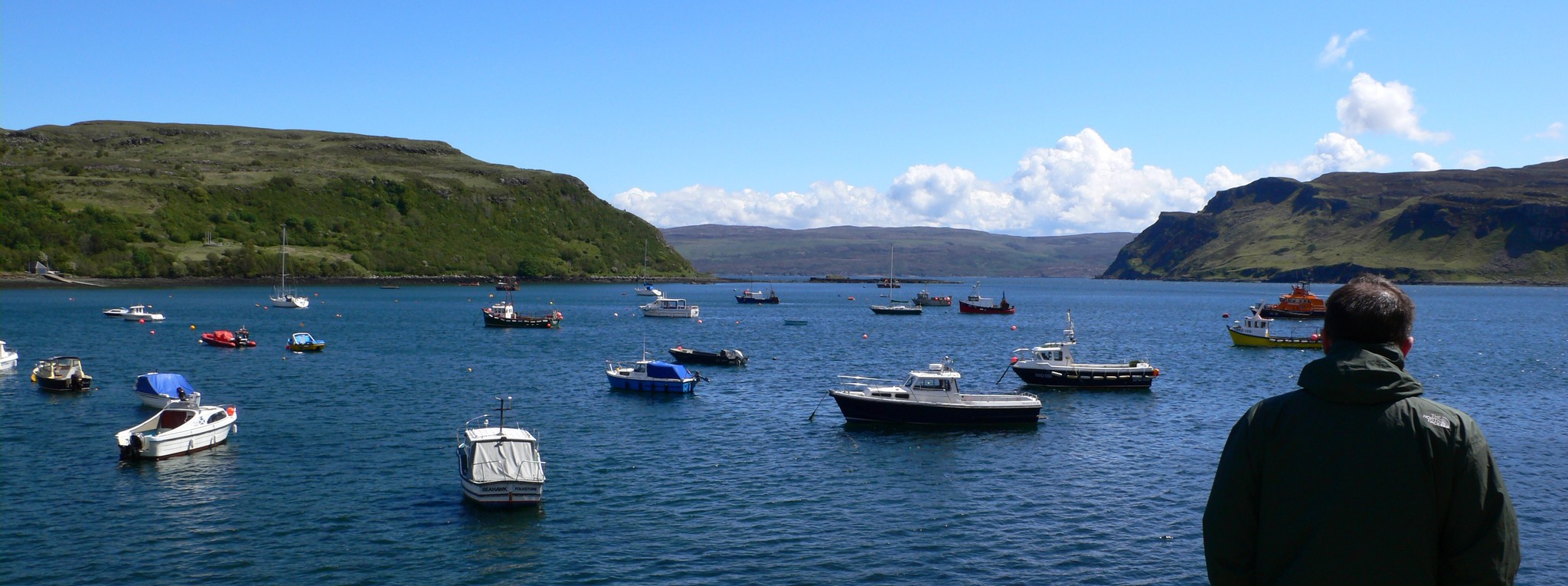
[
  {"x1": 634, "y1": 239, "x2": 662, "y2": 296},
  {"x1": 734, "y1": 270, "x2": 780, "y2": 304},
  {"x1": 269, "y1": 226, "x2": 311, "y2": 308}
]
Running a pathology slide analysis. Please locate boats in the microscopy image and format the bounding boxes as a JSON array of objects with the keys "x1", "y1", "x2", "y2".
[
  {"x1": 482, "y1": 277, "x2": 564, "y2": 328},
  {"x1": 119, "y1": 305, "x2": 167, "y2": 322},
  {"x1": 113, "y1": 402, "x2": 238, "y2": 459},
  {"x1": 460, "y1": 280, "x2": 480, "y2": 286},
  {"x1": 825, "y1": 357, "x2": 1054, "y2": 437},
  {"x1": 380, "y1": 285, "x2": 400, "y2": 289},
  {"x1": 957, "y1": 273, "x2": 1016, "y2": 314},
  {"x1": 996, "y1": 308, "x2": 1162, "y2": 389},
  {"x1": 31, "y1": 356, "x2": 92, "y2": 392},
  {"x1": 286, "y1": 333, "x2": 325, "y2": 354},
  {"x1": 199, "y1": 323, "x2": 257, "y2": 349},
  {"x1": 870, "y1": 244, "x2": 953, "y2": 315},
  {"x1": 132, "y1": 368, "x2": 202, "y2": 408},
  {"x1": 0, "y1": 341, "x2": 19, "y2": 371},
  {"x1": 495, "y1": 280, "x2": 522, "y2": 292},
  {"x1": 668, "y1": 346, "x2": 748, "y2": 367},
  {"x1": 638, "y1": 292, "x2": 700, "y2": 317},
  {"x1": 784, "y1": 320, "x2": 808, "y2": 325},
  {"x1": 103, "y1": 307, "x2": 131, "y2": 316},
  {"x1": 455, "y1": 395, "x2": 548, "y2": 505},
  {"x1": 604, "y1": 331, "x2": 711, "y2": 394},
  {"x1": 1220, "y1": 266, "x2": 1325, "y2": 353}
]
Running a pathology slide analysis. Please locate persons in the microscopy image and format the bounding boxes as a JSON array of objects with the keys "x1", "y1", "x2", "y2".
[{"x1": 1203, "y1": 273, "x2": 1522, "y2": 586}]
